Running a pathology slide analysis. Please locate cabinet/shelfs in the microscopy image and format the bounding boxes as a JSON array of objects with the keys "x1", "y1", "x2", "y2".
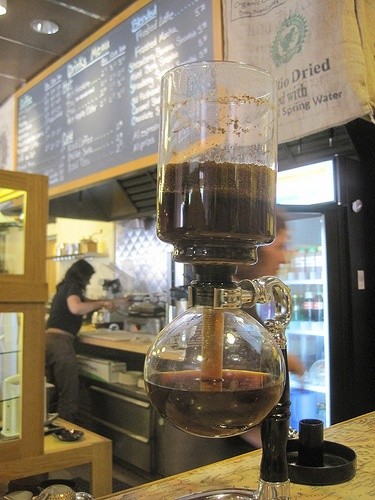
[{"x1": 0, "y1": 168, "x2": 48, "y2": 462}]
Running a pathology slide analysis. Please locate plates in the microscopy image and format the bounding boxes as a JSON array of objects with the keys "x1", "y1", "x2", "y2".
[{"x1": 53, "y1": 429, "x2": 83, "y2": 440}]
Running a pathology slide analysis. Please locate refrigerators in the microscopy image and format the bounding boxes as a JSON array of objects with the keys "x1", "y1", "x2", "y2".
[{"x1": 250, "y1": 157, "x2": 375, "y2": 451}]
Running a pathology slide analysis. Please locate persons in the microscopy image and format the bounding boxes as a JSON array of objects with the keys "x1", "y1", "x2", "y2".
[
  {"x1": 46, "y1": 260, "x2": 115, "y2": 424},
  {"x1": 227, "y1": 217, "x2": 306, "y2": 458}
]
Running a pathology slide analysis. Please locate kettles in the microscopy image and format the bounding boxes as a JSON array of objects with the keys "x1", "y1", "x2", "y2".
[{"x1": 2, "y1": 374, "x2": 47, "y2": 437}]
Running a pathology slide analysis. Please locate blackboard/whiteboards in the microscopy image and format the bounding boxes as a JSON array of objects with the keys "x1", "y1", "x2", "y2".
[{"x1": 7, "y1": 1, "x2": 226, "y2": 206}]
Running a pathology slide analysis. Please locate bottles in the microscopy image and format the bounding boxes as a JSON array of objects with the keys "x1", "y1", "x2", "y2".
[
  {"x1": 261, "y1": 286, "x2": 324, "y2": 322},
  {"x1": 278, "y1": 246, "x2": 322, "y2": 280}
]
